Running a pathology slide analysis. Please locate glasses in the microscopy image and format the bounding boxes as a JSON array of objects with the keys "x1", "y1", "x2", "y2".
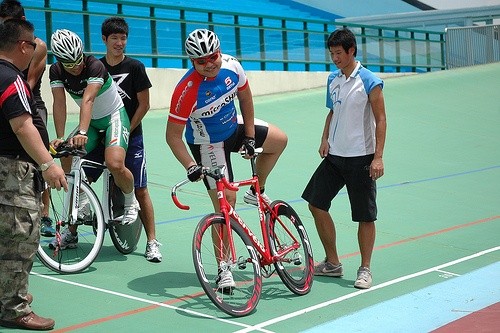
[
  {"x1": 60, "y1": 53, "x2": 83, "y2": 69},
  {"x1": 16, "y1": 39, "x2": 37, "y2": 51},
  {"x1": 192, "y1": 49, "x2": 219, "y2": 66}
]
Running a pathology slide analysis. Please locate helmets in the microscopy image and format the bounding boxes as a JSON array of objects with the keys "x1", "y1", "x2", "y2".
[
  {"x1": 185, "y1": 29, "x2": 221, "y2": 60},
  {"x1": 50, "y1": 29, "x2": 83, "y2": 63}
]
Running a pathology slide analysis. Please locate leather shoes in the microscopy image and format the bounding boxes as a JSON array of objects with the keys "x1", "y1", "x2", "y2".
[
  {"x1": 0, "y1": 310, "x2": 55, "y2": 331},
  {"x1": 27, "y1": 294, "x2": 33, "y2": 307}
]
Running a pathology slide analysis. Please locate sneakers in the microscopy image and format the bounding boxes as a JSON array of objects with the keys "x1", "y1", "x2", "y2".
[
  {"x1": 39, "y1": 216, "x2": 56, "y2": 238},
  {"x1": 353, "y1": 266, "x2": 374, "y2": 290},
  {"x1": 121, "y1": 198, "x2": 140, "y2": 226},
  {"x1": 48, "y1": 228, "x2": 79, "y2": 252},
  {"x1": 215, "y1": 261, "x2": 236, "y2": 292},
  {"x1": 78, "y1": 192, "x2": 94, "y2": 213},
  {"x1": 145, "y1": 239, "x2": 163, "y2": 263},
  {"x1": 302, "y1": 257, "x2": 344, "y2": 277},
  {"x1": 243, "y1": 184, "x2": 278, "y2": 210}
]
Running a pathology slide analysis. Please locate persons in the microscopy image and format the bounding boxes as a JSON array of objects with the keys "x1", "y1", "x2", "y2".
[
  {"x1": 47, "y1": 16, "x2": 165, "y2": 262},
  {"x1": 164, "y1": 27, "x2": 289, "y2": 291},
  {"x1": 0, "y1": 0, "x2": 58, "y2": 237},
  {"x1": 48, "y1": 27, "x2": 139, "y2": 228},
  {"x1": 300, "y1": 26, "x2": 387, "y2": 289},
  {"x1": 0, "y1": 15, "x2": 69, "y2": 329}
]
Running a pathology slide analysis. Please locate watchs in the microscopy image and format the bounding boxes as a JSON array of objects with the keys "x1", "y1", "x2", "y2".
[
  {"x1": 38, "y1": 160, "x2": 56, "y2": 174},
  {"x1": 76, "y1": 129, "x2": 88, "y2": 136}
]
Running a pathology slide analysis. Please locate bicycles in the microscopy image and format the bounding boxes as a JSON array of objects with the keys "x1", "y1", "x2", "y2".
[
  {"x1": 35, "y1": 140, "x2": 144, "y2": 274},
  {"x1": 170, "y1": 144, "x2": 314, "y2": 317}
]
